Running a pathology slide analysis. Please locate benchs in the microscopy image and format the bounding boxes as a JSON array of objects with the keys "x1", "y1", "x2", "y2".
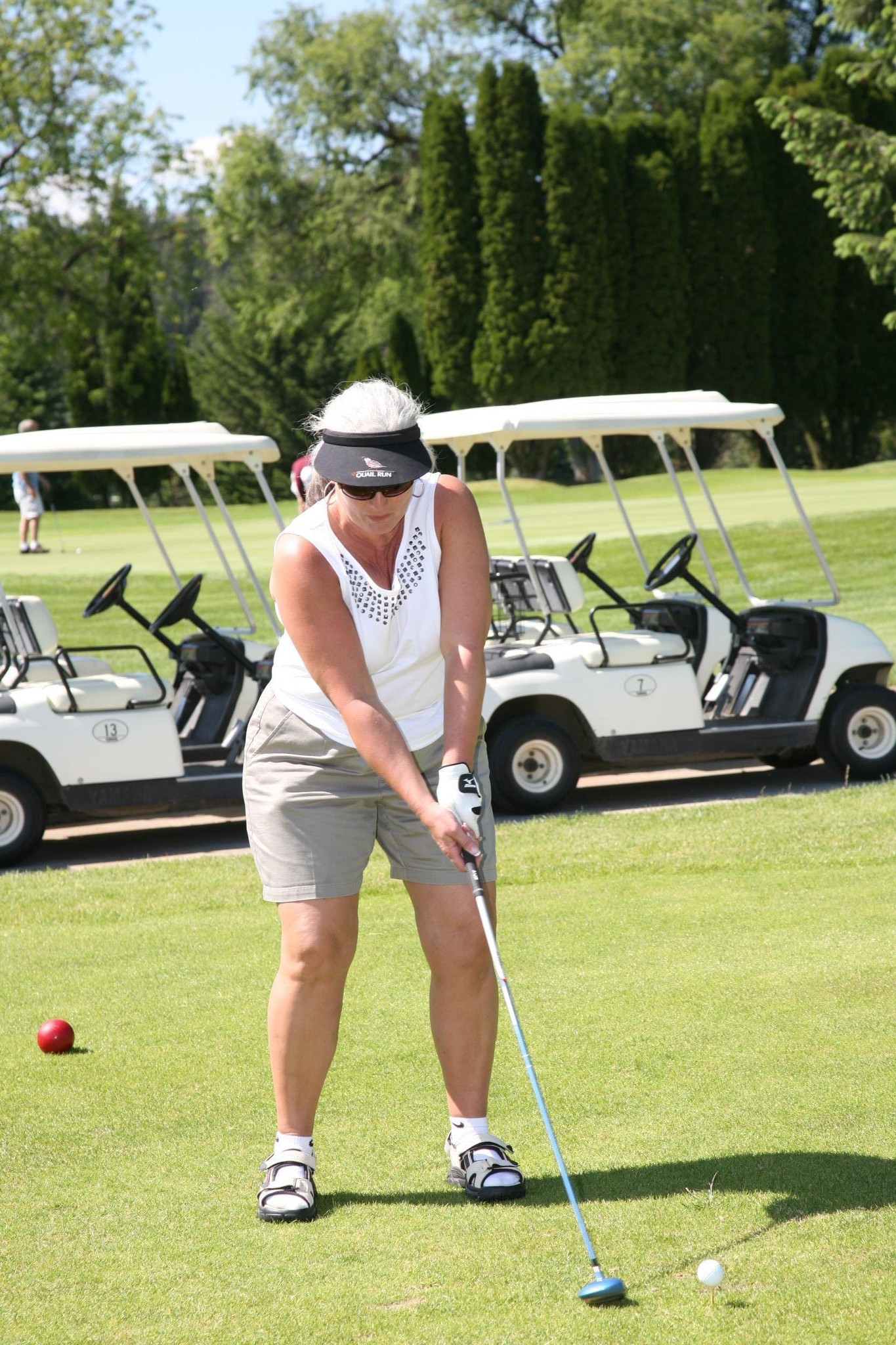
[
  {"x1": 484, "y1": 554, "x2": 698, "y2": 667},
  {"x1": 0, "y1": 591, "x2": 176, "y2": 713}
]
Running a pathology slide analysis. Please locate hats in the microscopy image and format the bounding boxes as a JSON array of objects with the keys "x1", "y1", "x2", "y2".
[{"x1": 314, "y1": 424, "x2": 432, "y2": 482}]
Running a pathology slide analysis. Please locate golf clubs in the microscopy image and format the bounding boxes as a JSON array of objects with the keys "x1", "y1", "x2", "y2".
[
  {"x1": 48, "y1": 490, "x2": 65, "y2": 552},
  {"x1": 460, "y1": 845, "x2": 628, "y2": 1306}
]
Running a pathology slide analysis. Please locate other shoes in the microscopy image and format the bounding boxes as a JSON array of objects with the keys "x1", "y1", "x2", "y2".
[
  {"x1": 29, "y1": 544, "x2": 49, "y2": 553},
  {"x1": 21, "y1": 546, "x2": 30, "y2": 553}
]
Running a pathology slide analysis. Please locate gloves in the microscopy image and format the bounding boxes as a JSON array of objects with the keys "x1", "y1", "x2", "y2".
[{"x1": 435, "y1": 761, "x2": 485, "y2": 842}]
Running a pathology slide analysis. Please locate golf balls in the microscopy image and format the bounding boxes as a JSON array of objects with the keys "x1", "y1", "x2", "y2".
[
  {"x1": 76, "y1": 547, "x2": 81, "y2": 554},
  {"x1": 696, "y1": 1259, "x2": 722, "y2": 1285}
]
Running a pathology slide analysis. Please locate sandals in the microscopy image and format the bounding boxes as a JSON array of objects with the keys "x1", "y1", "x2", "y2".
[
  {"x1": 443, "y1": 1133, "x2": 529, "y2": 1201},
  {"x1": 256, "y1": 1147, "x2": 319, "y2": 1220}
]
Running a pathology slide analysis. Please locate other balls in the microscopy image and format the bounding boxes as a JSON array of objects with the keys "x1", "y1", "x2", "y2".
[{"x1": 36, "y1": 1018, "x2": 75, "y2": 1055}]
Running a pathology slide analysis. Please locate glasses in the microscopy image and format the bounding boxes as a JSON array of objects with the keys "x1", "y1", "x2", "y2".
[{"x1": 337, "y1": 485, "x2": 416, "y2": 502}]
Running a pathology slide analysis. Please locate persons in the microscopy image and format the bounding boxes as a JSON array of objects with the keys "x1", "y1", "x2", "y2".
[
  {"x1": 290, "y1": 453, "x2": 315, "y2": 512},
  {"x1": 11, "y1": 418, "x2": 48, "y2": 553},
  {"x1": 242, "y1": 380, "x2": 528, "y2": 1220}
]
